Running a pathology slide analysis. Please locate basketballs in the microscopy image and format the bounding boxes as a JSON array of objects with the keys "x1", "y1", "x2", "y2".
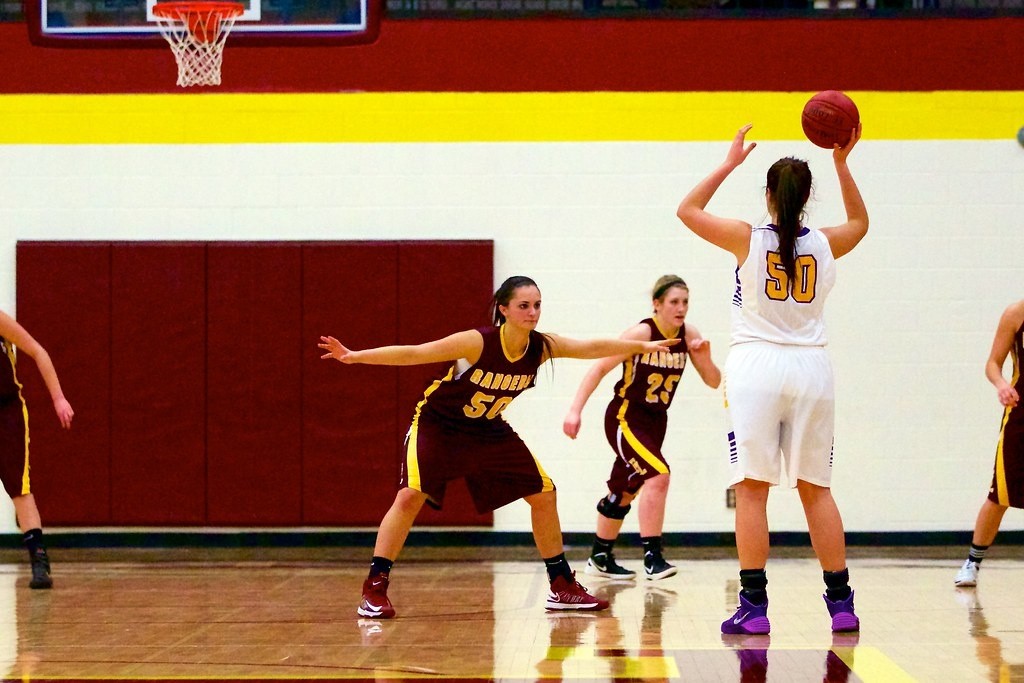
[{"x1": 801, "y1": 90, "x2": 860, "y2": 149}]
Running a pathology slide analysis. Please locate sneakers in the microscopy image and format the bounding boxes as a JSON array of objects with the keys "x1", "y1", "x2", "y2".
[
  {"x1": 641, "y1": 550, "x2": 678, "y2": 582},
  {"x1": 720, "y1": 593, "x2": 770, "y2": 633},
  {"x1": 822, "y1": 589, "x2": 859, "y2": 629},
  {"x1": 585, "y1": 550, "x2": 636, "y2": 580},
  {"x1": 545, "y1": 570, "x2": 609, "y2": 611},
  {"x1": 28, "y1": 554, "x2": 52, "y2": 589},
  {"x1": 358, "y1": 571, "x2": 396, "y2": 619},
  {"x1": 954, "y1": 558, "x2": 980, "y2": 586}
]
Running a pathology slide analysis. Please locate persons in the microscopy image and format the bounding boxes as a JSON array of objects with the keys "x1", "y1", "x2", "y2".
[
  {"x1": 317, "y1": 277, "x2": 681, "y2": 620},
  {"x1": 0, "y1": 311, "x2": 74, "y2": 587},
  {"x1": 678, "y1": 120, "x2": 869, "y2": 635},
  {"x1": 953, "y1": 296, "x2": 1023, "y2": 586},
  {"x1": 563, "y1": 274, "x2": 721, "y2": 579}
]
